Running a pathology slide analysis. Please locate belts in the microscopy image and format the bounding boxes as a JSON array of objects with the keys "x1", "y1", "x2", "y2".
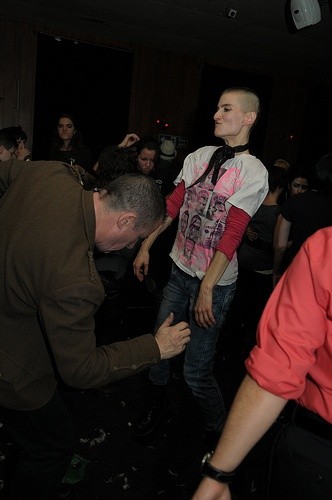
[{"x1": 284, "y1": 400, "x2": 332, "y2": 441}]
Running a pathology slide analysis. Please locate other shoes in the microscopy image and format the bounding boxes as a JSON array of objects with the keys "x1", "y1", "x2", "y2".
[
  {"x1": 168, "y1": 443, "x2": 214, "y2": 478},
  {"x1": 137, "y1": 397, "x2": 174, "y2": 431}
]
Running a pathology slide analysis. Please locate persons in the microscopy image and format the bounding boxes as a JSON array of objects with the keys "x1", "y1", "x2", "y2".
[
  {"x1": 190, "y1": 224, "x2": 331, "y2": 500},
  {"x1": 132, "y1": 86, "x2": 271, "y2": 454},
  {"x1": 1, "y1": 157, "x2": 193, "y2": 499},
  {"x1": 0, "y1": 113, "x2": 328, "y2": 398}
]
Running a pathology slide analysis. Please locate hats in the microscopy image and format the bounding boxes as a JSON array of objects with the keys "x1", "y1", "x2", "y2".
[{"x1": 159, "y1": 140, "x2": 178, "y2": 161}]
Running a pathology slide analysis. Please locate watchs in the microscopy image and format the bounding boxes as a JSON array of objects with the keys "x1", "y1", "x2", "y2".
[{"x1": 198, "y1": 450, "x2": 236, "y2": 486}]
